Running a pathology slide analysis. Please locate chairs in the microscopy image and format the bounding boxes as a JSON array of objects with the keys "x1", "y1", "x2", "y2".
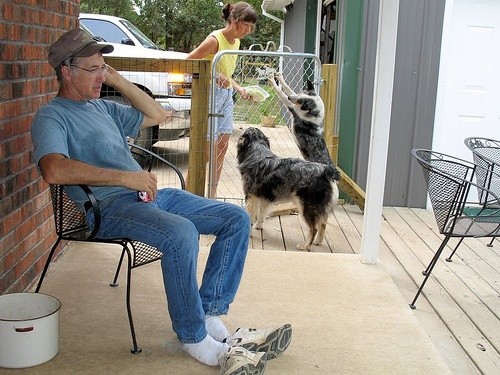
[
  {"x1": 408, "y1": 137, "x2": 500, "y2": 309},
  {"x1": 35, "y1": 144, "x2": 185, "y2": 357}
]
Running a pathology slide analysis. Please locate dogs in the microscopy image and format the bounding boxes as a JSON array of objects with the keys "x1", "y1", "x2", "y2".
[
  {"x1": 236, "y1": 126, "x2": 341, "y2": 249},
  {"x1": 266, "y1": 70, "x2": 335, "y2": 166}
]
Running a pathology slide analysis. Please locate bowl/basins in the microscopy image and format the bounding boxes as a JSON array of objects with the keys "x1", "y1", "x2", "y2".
[{"x1": 242, "y1": 85, "x2": 270, "y2": 101}]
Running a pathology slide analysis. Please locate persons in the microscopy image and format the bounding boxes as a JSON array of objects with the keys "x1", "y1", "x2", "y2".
[
  {"x1": 31, "y1": 28, "x2": 251, "y2": 366},
  {"x1": 185, "y1": 1, "x2": 259, "y2": 200}
]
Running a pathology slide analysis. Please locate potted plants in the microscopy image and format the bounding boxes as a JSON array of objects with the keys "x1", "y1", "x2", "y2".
[{"x1": 259, "y1": 104, "x2": 276, "y2": 127}]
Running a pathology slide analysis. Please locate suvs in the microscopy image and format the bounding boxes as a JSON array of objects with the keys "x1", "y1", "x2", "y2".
[{"x1": 77, "y1": 14, "x2": 196, "y2": 165}]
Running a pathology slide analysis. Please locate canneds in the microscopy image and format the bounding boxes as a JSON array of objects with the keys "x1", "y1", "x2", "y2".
[{"x1": 136, "y1": 190, "x2": 152, "y2": 202}]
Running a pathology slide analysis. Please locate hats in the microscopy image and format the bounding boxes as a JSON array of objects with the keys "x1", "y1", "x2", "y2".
[{"x1": 48, "y1": 28, "x2": 114, "y2": 68}]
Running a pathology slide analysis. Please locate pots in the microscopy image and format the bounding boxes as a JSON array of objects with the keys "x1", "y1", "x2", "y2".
[{"x1": 0, "y1": 292, "x2": 61, "y2": 369}]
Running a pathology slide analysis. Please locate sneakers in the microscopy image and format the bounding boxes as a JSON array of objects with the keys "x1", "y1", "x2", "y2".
[
  {"x1": 217, "y1": 346, "x2": 267, "y2": 375},
  {"x1": 223, "y1": 324, "x2": 292, "y2": 361}
]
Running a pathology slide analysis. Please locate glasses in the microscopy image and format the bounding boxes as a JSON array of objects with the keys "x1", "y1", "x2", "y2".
[{"x1": 62, "y1": 64, "x2": 110, "y2": 77}]
